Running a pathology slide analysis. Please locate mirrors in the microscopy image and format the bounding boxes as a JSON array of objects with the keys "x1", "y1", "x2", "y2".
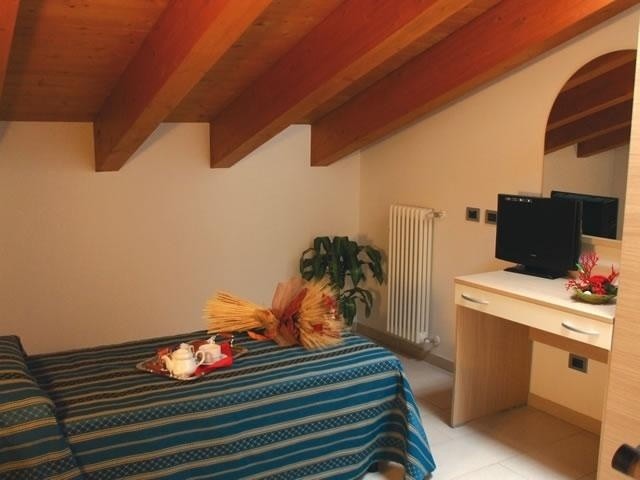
[{"x1": 536, "y1": 50, "x2": 637, "y2": 243}]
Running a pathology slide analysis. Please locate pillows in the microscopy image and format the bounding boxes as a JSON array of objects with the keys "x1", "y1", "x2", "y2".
[{"x1": 0, "y1": 335, "x2": 81, "y2": 480}]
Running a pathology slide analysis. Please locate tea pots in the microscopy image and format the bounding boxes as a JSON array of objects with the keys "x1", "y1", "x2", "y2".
[{"x1": 160, "y1": 347, "x2": 204, "y2": 379}]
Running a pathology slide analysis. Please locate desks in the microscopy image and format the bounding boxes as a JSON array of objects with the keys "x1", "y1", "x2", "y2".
[{"x1": 448, "y1": 271, "x2": 617, "y2": 462}]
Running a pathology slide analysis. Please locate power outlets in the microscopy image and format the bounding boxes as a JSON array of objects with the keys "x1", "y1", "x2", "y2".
[
  {"x1": 467, "y1": 207, "x2": 480, "y2": 223},
  {"x1": 567, "y1": 353, "x2": 588, "y2": 375},
  {"x1": 484, "y1": 209, "x2": 498, "y2": 224}
]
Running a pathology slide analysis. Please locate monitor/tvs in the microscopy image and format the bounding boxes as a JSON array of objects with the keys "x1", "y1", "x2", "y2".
[
  {"x1": 494, "y1": 193, "x2": 579, "y2": 280},
  {"x1": 550, "y1": 189, "x2": 619, "y2": 241}
]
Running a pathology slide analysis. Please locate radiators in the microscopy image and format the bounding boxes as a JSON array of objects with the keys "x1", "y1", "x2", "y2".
[{"x1": 385, "y1": 203, "x2": 436, "y2": 348}]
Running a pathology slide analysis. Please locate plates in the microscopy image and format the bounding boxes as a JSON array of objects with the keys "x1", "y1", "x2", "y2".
[
  {"x1": 573, "y1": 286, "x2": 615, "y2": 304},
  {"x1": 198, "y1": 353, "x2": 228, "y2": 365},
  {"x1": 135, "y1": 339, "x2": 248, "y2": 382}
]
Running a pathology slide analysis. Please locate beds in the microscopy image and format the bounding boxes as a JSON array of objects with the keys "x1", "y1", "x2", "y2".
[{"x1": 0, "y1": 324, "x2": 439, "y2": 480}]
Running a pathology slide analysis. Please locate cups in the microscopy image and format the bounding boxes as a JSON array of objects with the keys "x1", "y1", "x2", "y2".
[{"x1": 198, "y1": 344, "x2": 220, "y2": 362}]
